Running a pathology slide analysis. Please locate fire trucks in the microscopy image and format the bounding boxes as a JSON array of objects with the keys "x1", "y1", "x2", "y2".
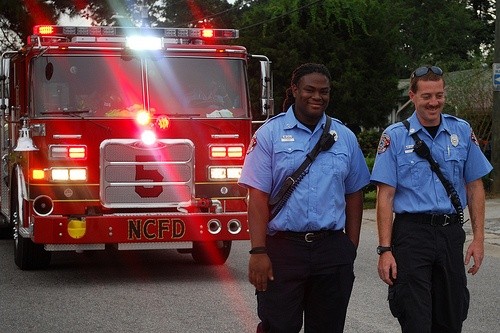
[{"x1": 0, "y1": 25, "x2": 275, "y2": 271}]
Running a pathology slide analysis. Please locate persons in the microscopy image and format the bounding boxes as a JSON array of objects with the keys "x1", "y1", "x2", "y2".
[
  {"x1": 180, "y1": 74, "x2": 233, "y2": 111},
  {"x1": 370, "y1": 65, "x2": 493, "y2": 333},
  {"x1": 237, "y1": 63, "x2": 371, "y2": 333}
]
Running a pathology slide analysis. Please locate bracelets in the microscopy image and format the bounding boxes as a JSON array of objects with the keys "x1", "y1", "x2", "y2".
[
  {"x1": 223, "y1": 93, "x2": 228, "y2": 98},
  {"x1": 248, "y1": 246, "x2": 270, "y2": 255}
]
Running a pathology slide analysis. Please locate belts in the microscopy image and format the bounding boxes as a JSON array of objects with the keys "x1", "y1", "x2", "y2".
[
  {"x1": 272, "y1": 229, "x2": 344, "y2": 242},
  {"x1": 395, "y1": 213, "x2": 459, "y2": 226}
]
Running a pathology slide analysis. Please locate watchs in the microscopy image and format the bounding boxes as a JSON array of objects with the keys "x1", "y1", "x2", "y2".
[{"x1": 377, "y1": 245, "x2": 393, "y2": 255}]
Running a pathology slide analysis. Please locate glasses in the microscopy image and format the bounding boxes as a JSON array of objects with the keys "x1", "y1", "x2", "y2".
[{"x1": 411, "y1": 66, "x2": 444, "y2": 83}]
